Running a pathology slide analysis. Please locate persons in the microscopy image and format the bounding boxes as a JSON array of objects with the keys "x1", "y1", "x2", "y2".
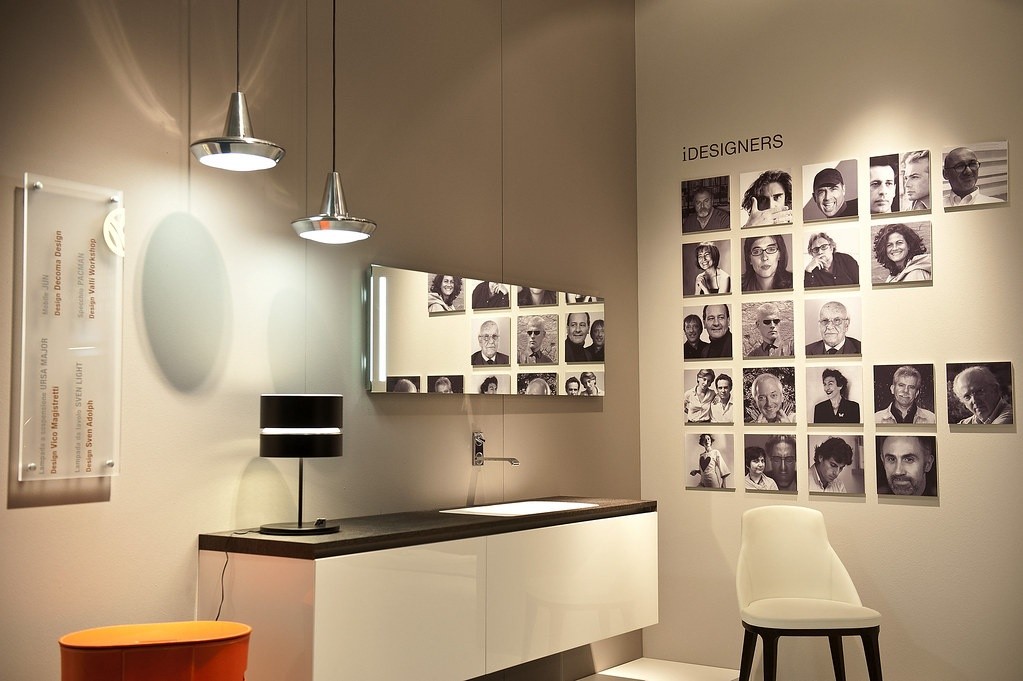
[
  {"x1": 811, "y1": 169, "x2": 857, "y2": 219},
  {"x1": 805, "y1": 300, "x2": 861, "y2": 356},
  {"x1": 812, "y1": 368, "x2": 860, "y2": 424},
  {"x1": 808, "y1": 436, "x2": 853, "y2": 495},
  {"x1": 681, "y1": 187, "x2": 728, "y2": 232},
  {"x1": 803, "y1": 233, "x2": 859, "y2": 289},
  {"x1": 739, "y1": 169, "x2": 793, "y2": 228},
  {"x1": 746, "y1": 303, "x2": 794, "y2": 358},
  {"x1": 752, "y1": 372, "x2": 796, "y2": 425},
  {"x1": 870, "y1": 150, "x2": 932, "y2": 216},
  {"x1": 690, "y1": 434, "x2": 731, "y2": 489},
  {"x1": 943, "y1": 147, "x2": 1006, "y2": 207},
  {"x1": 385, "y1": 274, "x2": 604, "y2": 396},
  {"x1": 874, "y1": 224, "x2": 932, "y2": 283},
  {"x1": 683, "y1": 304, "x2": 732, "y2": 358},
  {"x1": 874, "y1": 366, "x2": 936, "y2": 426},
  {"x1": 951, "y1": 366, "x2": 1013, "y2": 424},
  {"x1": 879, "y1": 435, "x2": 934, "y2": 496},
  {"x1": 743, "y1": 434, "x2": 797, "y2": 493},
  {"x1": 695, "y1": 241, "x2": 731, "y2": 296},
  {"x1": 683, "y1": 369, "x2": 735, "y2": 424},
  {"x1": 742, "y1": 235, "x2": 793, "y2": 292}
]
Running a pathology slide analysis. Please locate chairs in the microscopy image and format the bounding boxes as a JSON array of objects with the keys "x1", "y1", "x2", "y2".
[{"x1": 736, "y1": 505, "x2": 886, "y2": 681}]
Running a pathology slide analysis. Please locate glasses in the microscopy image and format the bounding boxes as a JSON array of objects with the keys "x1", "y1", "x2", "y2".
[
  {"x1": 749, "y1": 246, "x2": 779, "y2": 256},
  {"x1": 758, "y1": 319, "x2": 781, "y2": 323},
  {"x1": 527, "y1": 330, "x2": 545, "y2": 335},
  {"x1": 480, "y1": 334, "x2": 499, "y2": 341},
  {"x1": 945, "y1": 159, "x2": 981, "y2": 172},
  {"x1": 819, "y1": 317, "x2": 847, "y2": 324},
  {"x1": 811, "y1": 243, "x2": 831, "y2": 253},
  {"x1": 960, "y1": 385, "x2": 994, "y2": 401}
]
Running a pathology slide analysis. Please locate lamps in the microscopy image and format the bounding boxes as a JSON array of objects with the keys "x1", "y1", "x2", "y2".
[
  {"x1": 189, "y1": 1, "x2": 285, "y2": 173},
  {"x1": 290, "y1": 1, "x2": 377, "y2": 245},
  {"x1": 257, "y1": 394, "x2": 344, "y2": 536}
]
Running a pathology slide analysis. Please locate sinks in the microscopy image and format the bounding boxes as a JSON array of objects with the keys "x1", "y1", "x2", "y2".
[{"x1": 438, "y1": 500, "x2": 600, "y2": 517}]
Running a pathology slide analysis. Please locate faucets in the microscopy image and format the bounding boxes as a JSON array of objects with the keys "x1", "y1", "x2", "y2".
[{"x1": 477, "y1": 456, "x2": 521, "y2": 467}]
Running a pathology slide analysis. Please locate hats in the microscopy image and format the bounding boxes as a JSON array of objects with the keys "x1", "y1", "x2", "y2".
[{"x1": 813, "y1": 168, "x2": 844, "y2": 191}]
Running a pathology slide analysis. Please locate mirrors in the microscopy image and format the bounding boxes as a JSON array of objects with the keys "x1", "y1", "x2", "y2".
[{"x1": 367, "y1": 262, "x2": 607, "y2": 398}]
